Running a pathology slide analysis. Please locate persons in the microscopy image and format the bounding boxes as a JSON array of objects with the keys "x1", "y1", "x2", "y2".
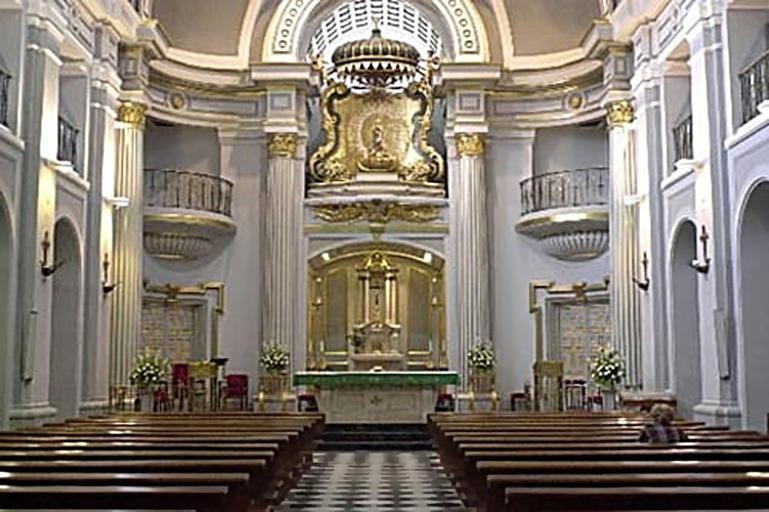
[
  {"x1": 635, "y1": 404, "x2": 688, "y2": 441},
  {"x1": 134, "y1": 392, "x2": 141, "y2": 411},
  {"x1": 153, "y1": 384, "x2": 167, "y2": 412}
]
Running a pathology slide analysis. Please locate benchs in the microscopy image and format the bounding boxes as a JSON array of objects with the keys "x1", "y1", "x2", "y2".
[
  {"x1": 424, "y1": 412, "x2": 768, "y2": 511},
  {"x1": 0, "y1": 411, "x2": 326, "y2": 512}
]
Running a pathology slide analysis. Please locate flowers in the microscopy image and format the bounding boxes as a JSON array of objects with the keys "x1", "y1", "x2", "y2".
[
  {"x1": 129, "y1": 351, "x2": 169, "y2": 385},
  {"x1": 259, "y1": 346, "x2": 289, "y2": 374},
  {"x1": 467, "y1": 338, "x2": 495, "y2": 370},
  {"x1": 591, "y1": 345, "x2": 626, "y2": 389}
]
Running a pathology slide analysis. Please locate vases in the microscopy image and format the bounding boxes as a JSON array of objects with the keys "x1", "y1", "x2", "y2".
[
  {"x1": 471, "y1": 370, "x2": 494, "y2": 393},
  {"x1": 262, "y1": 369, "x2": 288, "y2": 395}
]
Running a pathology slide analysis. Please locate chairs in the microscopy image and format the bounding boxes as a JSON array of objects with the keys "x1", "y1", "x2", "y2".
[{"x1": 218, "y1": 373, "x2": 248, "y2": 410}]
